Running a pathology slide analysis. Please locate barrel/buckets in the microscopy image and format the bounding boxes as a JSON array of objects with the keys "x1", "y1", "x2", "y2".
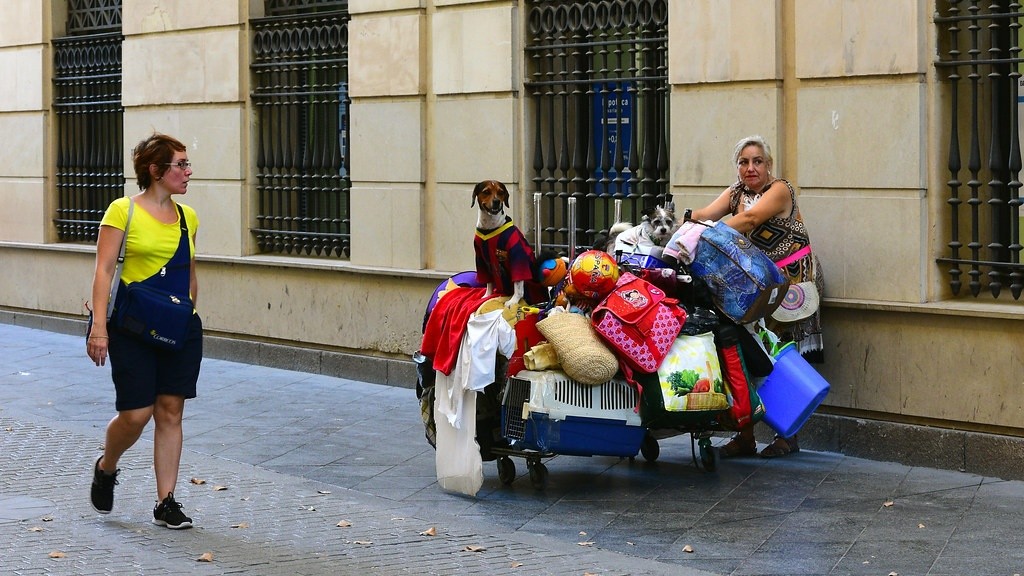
[{"x1": 754, "y1": 332, "x2": 831, "y2": 438}]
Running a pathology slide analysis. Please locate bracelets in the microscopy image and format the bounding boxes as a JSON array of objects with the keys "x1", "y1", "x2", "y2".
[{"x1": 90, "y1": 336, "x2": 107, "y2": 338}]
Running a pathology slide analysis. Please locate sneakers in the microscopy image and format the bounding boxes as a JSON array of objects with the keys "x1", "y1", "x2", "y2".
[
  {"x1": 91, "y1": 455, "x2": 120, "y2": 514},
  {"x1": 152, "y1": 492, "x2": 193, "y2": 528}
]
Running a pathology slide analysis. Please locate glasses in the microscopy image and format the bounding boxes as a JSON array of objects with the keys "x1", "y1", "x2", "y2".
[{"x1": 164, "y1": 161, "x2": 191, "y2": 170}]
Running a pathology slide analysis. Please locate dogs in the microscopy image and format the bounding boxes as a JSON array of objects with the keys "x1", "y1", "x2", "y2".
[
  {"x1": 469, "y1": 181, "x2": 553, "y2": 306},
  {"x1": 607, "y1": 203, "x2": 676, "y2": 260}
]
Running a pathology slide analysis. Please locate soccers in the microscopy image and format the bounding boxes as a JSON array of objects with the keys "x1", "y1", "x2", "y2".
[{"x1": 570, "y1": 249, "x2": 619, "y2": 300}]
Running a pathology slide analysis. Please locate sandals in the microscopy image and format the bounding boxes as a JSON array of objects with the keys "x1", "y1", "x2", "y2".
[
  {"x1": 761, "y1": 433, "x2": 799, "y2": 457},
  {"x1": 719, "y1": 432, "x2": 757, "y2": 457}
]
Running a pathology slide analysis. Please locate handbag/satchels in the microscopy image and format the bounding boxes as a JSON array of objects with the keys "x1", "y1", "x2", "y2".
[
  {"x1": 113, "y1": 282, "x2": 194, "y2": 352},
  {"x1": 771, "y1": 281, "x2": 819, "y2": 323},
  {"x1": 86, "y1": 310, "x2": 111, "y2": 346},
  {"x1": 538, "y1": 219, "x2": 790, "y2": 424}
]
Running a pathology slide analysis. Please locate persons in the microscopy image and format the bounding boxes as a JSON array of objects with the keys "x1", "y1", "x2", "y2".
[
  {"x1": 86, "y1": 134, "x2": 204, "y2": 528},
  {"x1": 678, "y1": 134, "x2": 826, "y2": 458}
]
